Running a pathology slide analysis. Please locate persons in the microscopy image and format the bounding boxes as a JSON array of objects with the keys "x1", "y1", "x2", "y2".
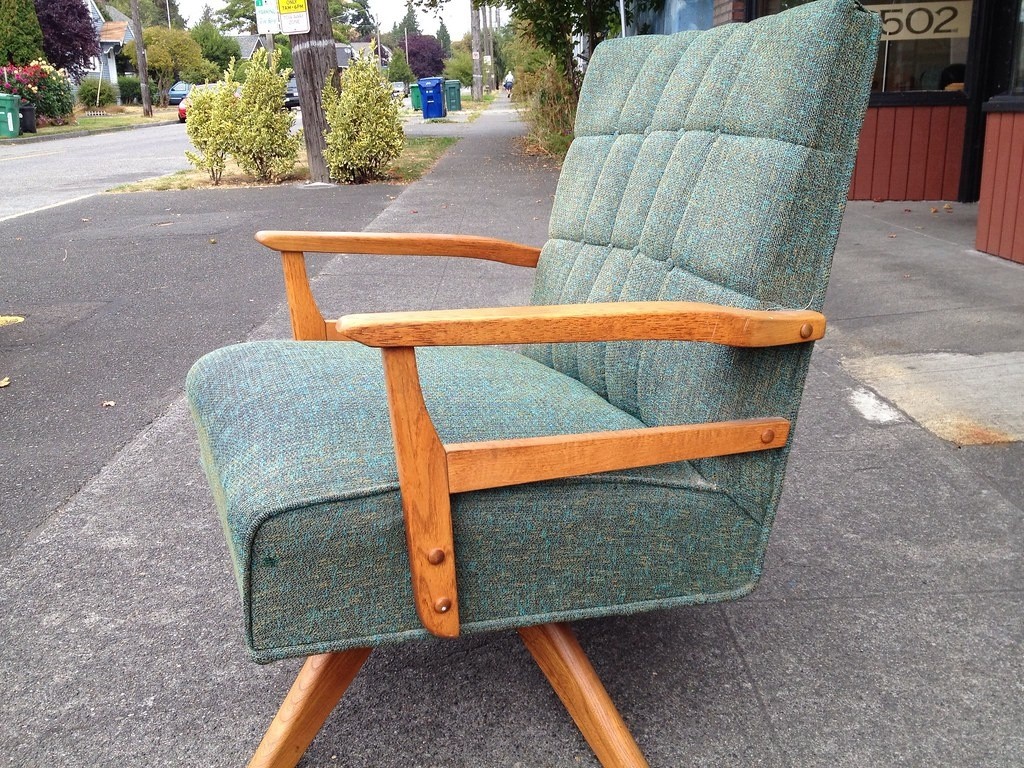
[{"x1": 504, "y1": 70, "x2": 515, "y2": 99}]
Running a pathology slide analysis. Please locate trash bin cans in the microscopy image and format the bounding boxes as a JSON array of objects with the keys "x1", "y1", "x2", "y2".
[
  {"x1": 21, "y1": 106, "x2": 37, "y2": 134},
  {"x1": 418, "y1": 77, "x2": 447, "y2": 118},
  {"x1": 0, "y1": 92, "x2": 22, "y2": 138},
  {"x1": 409, "y1": 84, "x2": 423, "y2": 110},
  {"x1": 445, "y1": 80, "x2": 462, "y2": 111}
]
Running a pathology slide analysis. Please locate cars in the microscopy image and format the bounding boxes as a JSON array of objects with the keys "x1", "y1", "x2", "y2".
[
  {"x1": 280, "y1": 77, "x2": 300, "y2": 111},
  {"x1": 391, "y1": 82, "x2": 407, "y2": 98},
  {"x1": 177, "y1": 83, "x2": 241, "y2": 124},
  {"x1": 168, "y1": 80, "x2": 193, "y2": 104}
]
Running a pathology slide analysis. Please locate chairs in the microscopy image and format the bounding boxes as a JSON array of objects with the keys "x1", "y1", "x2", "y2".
[{"x1": 183, "y1": 0, "x2": 880, "y2": 767}]
